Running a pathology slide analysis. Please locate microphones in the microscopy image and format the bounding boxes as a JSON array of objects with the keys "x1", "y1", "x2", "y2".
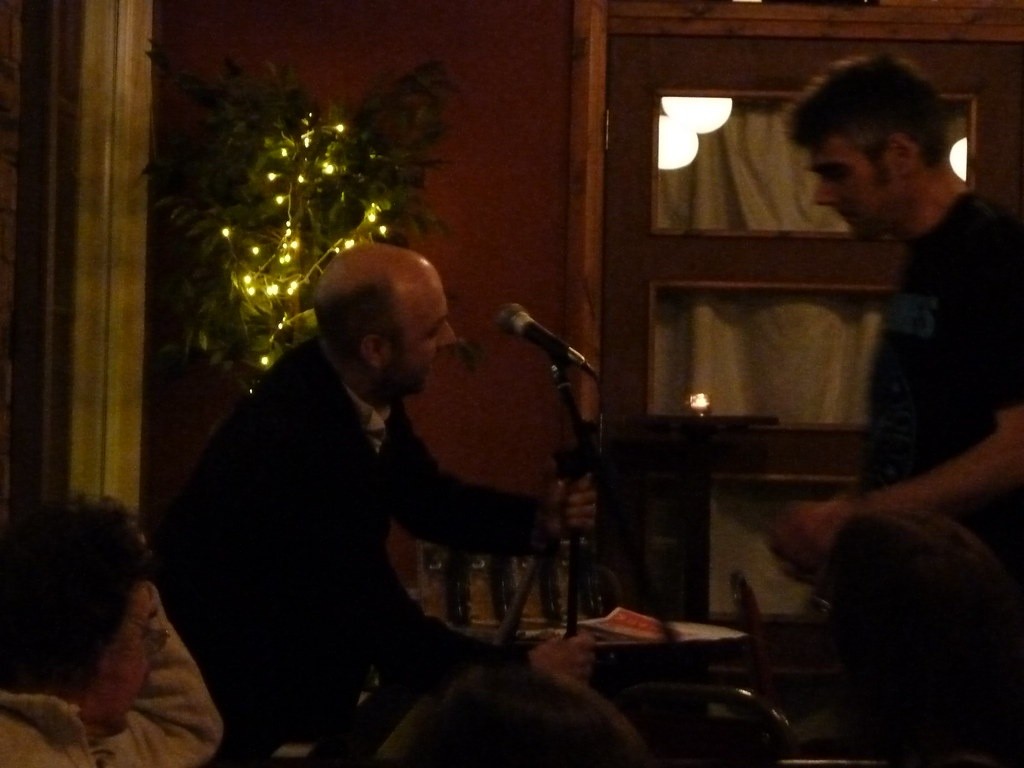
[{"x1": 499, "y1": 302, "x2": 600, "y2": 379}]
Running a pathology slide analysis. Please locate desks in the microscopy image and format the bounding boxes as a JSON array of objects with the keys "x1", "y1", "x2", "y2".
[{"x1": 452, "y1": 618, "x2": 751, "y2": 687}]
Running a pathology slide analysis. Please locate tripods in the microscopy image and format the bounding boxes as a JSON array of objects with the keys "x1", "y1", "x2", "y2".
[{"x1": 482, "y1": 365, "x2": 687, "y2": 642}]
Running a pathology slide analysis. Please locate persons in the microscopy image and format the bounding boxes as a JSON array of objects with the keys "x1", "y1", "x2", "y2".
[
  {"x1": 802, "y1": 504, "x2": 1024, "y2": 768},
  {"x1": 763, "y1": 52, "x2": 1024, "y2": 595},
  {"x1": 1, "y1": 488, "x2": 226, "y2": 767},
  {"x1": 146, "y1": 242, "x2": 601, "y2": 767},
  {"x1": 371, "y1": 665, "x2": 664, "y2": 767}
]
